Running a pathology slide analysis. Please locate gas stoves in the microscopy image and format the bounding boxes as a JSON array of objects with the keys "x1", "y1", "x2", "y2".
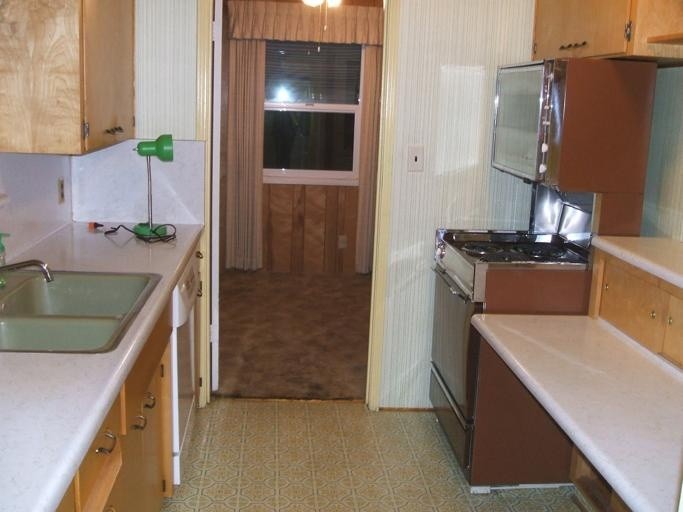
[{"x1": 442, "y1": 226, "x2": 580, "y2": 282}]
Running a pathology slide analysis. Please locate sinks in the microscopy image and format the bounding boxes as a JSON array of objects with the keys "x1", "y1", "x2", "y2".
[
  {"x1": 0, "y1": 270, "x2": 161, "y2": 318},
  {"x1": 0, "y1": 318, "x2": 123, "y2": 355}
]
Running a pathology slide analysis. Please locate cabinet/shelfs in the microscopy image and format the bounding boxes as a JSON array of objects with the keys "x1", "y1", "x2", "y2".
[
  {"x1": 0, "y1": 0, "x2": 132, "y2": 156},
  {"x1": 531, "y1": 1, "x2": 682, "y2": 62},
  {"x1": 586, "y1": 246, "x2": 683, "y2": 382},
  {"x1": 56, "y1": 290, "x2": 173, "y2": 512}
]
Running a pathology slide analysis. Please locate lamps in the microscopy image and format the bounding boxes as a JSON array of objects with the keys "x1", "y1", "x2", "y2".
[{"x1": 132, "y1": 135, "x2": 173, "y2": 239}]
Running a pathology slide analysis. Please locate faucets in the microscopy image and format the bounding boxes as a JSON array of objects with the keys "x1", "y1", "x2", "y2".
[{"x1": 0, "y1": 260, "x2": 54, "y2": 283}]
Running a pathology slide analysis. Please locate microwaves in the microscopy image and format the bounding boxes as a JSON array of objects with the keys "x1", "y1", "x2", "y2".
[{"x1": 487, "y1": 58, "x2": 564, "y2": 182}]
